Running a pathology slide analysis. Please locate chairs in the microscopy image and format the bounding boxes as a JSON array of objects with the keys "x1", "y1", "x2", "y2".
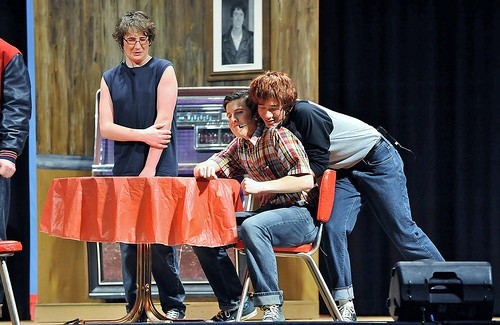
[
  {"x1": 0, "y1": 240, "x2": 23, "y2": 325},
  {"x1": 233, "y1": 168, "x2": 344, "y2": 322}
]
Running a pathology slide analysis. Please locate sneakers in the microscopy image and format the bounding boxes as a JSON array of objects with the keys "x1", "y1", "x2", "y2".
[
  {"x1": 336, "y1": 304, "x2": 357, "y2": 323},
  {"x1": 206, "y1": 298, "x2": 257, "y2": 322},
  {"x1": 164, "y1": 309, "x2": 187, "y2": 322},
  {"x1": 261, "y1": 304, "x2": 284, "y2": 322}
]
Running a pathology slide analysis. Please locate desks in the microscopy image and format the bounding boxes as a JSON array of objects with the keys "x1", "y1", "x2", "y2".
[{"x1": 38, "y1": 177, "x2": 246, "y2": 325}]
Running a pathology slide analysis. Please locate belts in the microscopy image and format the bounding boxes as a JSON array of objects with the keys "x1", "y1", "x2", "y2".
[{"x1": 355, "y1": 138, "x2": 384, "y2": 169}]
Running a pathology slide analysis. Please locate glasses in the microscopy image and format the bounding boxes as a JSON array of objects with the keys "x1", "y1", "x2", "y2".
[{"x1": 123, "y1": 36, "x2": 149, "y2": 44}]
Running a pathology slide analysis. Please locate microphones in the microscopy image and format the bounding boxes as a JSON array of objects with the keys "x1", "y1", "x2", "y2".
[
  {"x1": 239, "y1": 118, "x2": 252, "y2": 128},
  {"x1": 273, "y1": 107, "x2": 293, "y2": 126}
]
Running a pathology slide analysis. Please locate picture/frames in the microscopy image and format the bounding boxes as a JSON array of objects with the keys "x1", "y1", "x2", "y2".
[{"x1": 205, "y1": 0, "x2": 271, "y2": 83}]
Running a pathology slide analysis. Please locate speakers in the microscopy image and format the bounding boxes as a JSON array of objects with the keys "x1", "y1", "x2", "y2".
[{"x1": 386, "y1": 259, "x2": 494, "y2": 322}]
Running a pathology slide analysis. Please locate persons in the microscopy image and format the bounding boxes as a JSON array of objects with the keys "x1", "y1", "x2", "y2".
[
  {"x1": 99, "y1": 11, "x2": 186, "y2": 321},
  {"x1": 222, "y1": 3, "x2": 254, "y2": 63},
  {"x1": 248, "y1": 70, "x2": 445, "y2": 322},
  {"x1": 192, "y1": 90, "x2": 320, "y2": 321},
  {"x1": 0, "y1": 36, "x2": 32, "y2": 316}
]
